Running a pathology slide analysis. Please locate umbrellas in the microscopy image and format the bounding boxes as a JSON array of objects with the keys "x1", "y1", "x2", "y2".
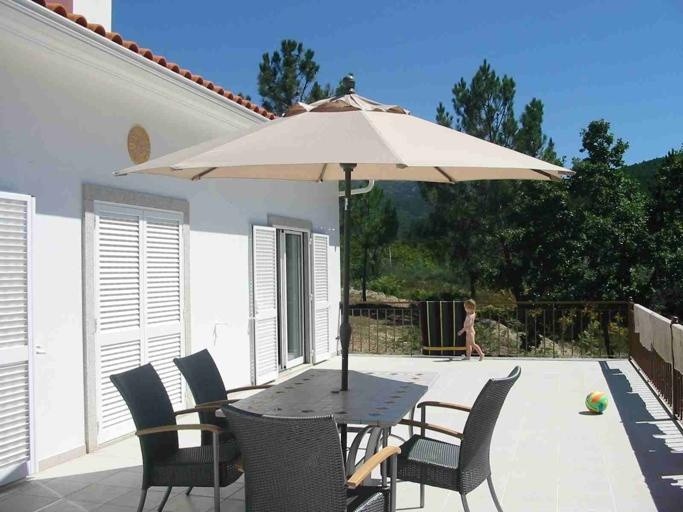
[{"x1": 104, "y1": 70, "x2": 577, "y2": 472}]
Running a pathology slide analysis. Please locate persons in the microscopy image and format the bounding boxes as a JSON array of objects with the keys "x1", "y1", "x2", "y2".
[{"x1": 455, "y1": 299, "x2": 484, "y2": 362}]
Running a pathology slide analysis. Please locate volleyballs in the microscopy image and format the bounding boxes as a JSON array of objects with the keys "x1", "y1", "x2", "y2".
[{"x1": 586, "y1": 392, "x2": 608, "y2": 413}]
